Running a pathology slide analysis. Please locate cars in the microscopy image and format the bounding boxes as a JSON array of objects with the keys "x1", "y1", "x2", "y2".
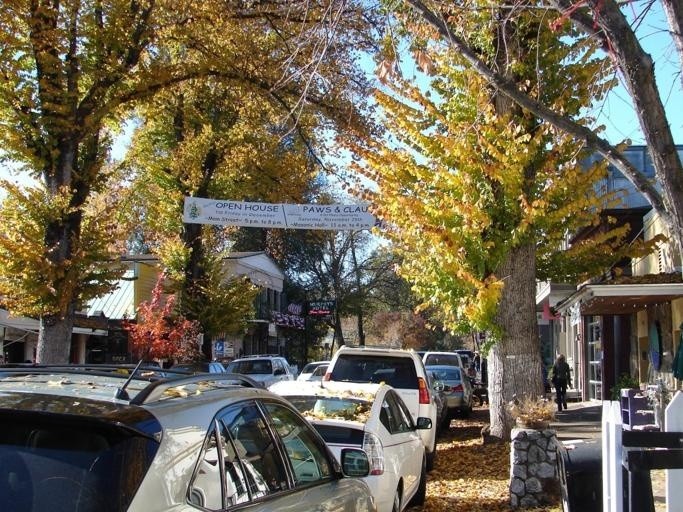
[
  {"x1": 269, "y1": 382, "x2": 433, "y2": 510},
  {"x1": 298, "y1": 360, "x2": 330, "y2": 382},
  {"x1": 424, "y1": 371, "x2": 449, "y2": 425},
  {"x1": 164, "y1": 362, "x2": 226, "y2": 381},
  {"x1": 425, "y1": 366, "x2": 476, "y2": 413},
  {"x1": 417, "y1": 349, "x2": 479, "y2": 386}
]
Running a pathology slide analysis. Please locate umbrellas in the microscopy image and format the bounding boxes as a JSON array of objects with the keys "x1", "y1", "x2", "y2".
[{"x1": 674, "y1": 320, "x2": 683, "y2": 392}]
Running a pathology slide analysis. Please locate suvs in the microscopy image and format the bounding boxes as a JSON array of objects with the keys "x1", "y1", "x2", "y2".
[
  {"x1": 319, "y1": 345, "x2": 445, "y2": 471},
  {"x1": 0, "y1": 360, "x2": 375, "y2": 512},
  {"x1": 224, "y1": 354, "x2": 296, "y2": 390}
]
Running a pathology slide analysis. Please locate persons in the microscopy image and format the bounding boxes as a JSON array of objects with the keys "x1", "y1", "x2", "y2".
[
  {"x1": 539, "y1": 361, "x2": 549, "y2": 391},
  {"x1": 468, "y1": 362, "x2": 477, "y2": 388},
  {"x1": 480, "y1": 357, "x2": 487, "y2": 383},
  {"x1": 0, "y1": 354, "x2": 4, "y2": 365},
  {"x1": 472, "y1": 351, "x2": 480, "y2": 371},
  {"x1": 552, "y1": 354, "x2": 572, "y2": 411}
]
